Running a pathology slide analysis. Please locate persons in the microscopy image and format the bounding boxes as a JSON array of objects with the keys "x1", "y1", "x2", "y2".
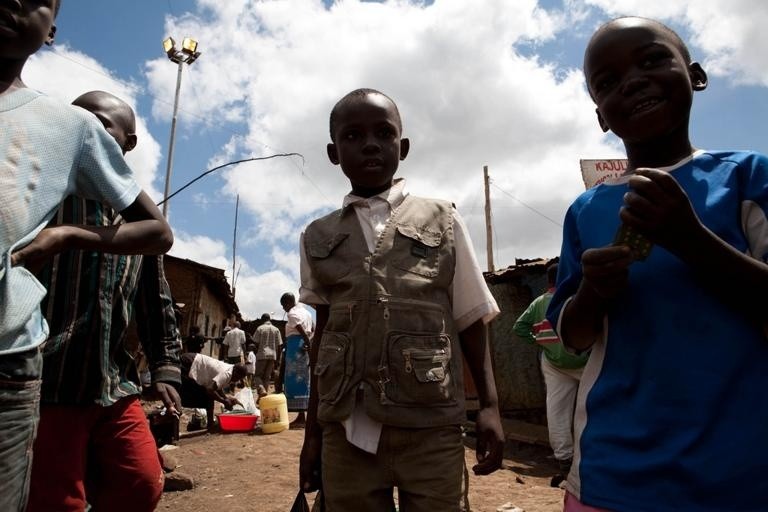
[
  {"x1": 20, "y1": 92, "x2": 182, "y2": 512},
  {"x1": 546, "y1": 16, "x2": 768, "y2": 512},
  {"x1": 514, "y1": 262, "x2": 586, "y2": 488},
  {"x1": 297, "y1": 88, "x2": 504, "y2": 512},
  {"x1": 181, "y1": 293, "x2": 313, "y2": 433},
  {"x1": 0, "y1": 0, "x2": 173, "y2": 512}
]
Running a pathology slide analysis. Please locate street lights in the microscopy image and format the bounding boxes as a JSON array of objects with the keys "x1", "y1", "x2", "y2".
[{"x1": 162, "y1": 37, "x2": 201, "y2": 222}]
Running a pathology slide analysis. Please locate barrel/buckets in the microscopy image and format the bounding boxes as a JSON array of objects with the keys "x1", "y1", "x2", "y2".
[{"x1": 259, "y1": 394, "x2": 289, "y2": 435}]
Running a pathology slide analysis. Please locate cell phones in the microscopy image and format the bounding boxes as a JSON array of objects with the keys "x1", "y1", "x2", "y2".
[{"x1": 615, "y1": 187, "x2": 658, "y2": 262}]
